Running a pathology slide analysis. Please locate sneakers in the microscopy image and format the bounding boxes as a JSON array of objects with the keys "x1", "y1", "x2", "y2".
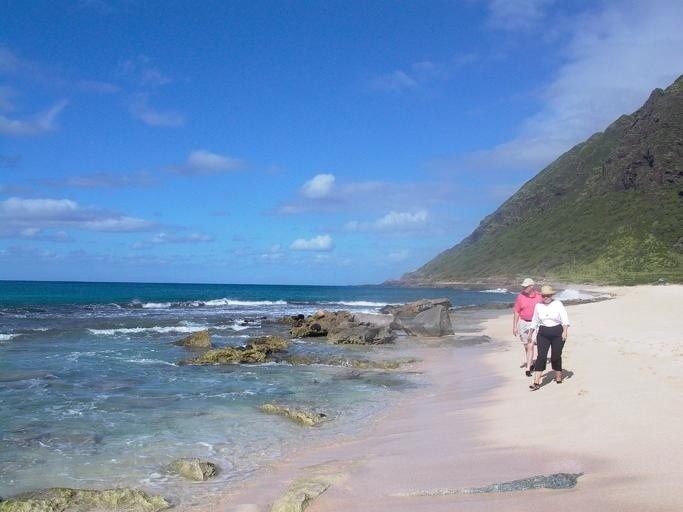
[{"x1": 529, "y1": 380, "x2": 562, "y2": 390}]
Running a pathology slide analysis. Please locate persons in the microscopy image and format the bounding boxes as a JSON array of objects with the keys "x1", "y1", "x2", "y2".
[
  {"x1": 528, "y1": 283, "x2": 569, "y2": 389},
  {"x1": 511, "y1": 277, "x2": 543, "y2": 376}
]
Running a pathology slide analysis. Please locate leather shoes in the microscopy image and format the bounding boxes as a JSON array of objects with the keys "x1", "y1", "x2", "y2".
[{"x1": 526, "y1": 363, "x2": 535, "y2": 377}]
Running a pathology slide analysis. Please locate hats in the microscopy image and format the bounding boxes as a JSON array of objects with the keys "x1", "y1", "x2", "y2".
[
  {"x1": 537, "y1": 285, "x2": 557, "y2": 295},
  {"x1": 520, "y1": 278, "x2": 534, "y2": 287}
]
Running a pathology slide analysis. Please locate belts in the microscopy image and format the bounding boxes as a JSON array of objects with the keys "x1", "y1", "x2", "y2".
[{"x1": 525, "y1": 320, "x2": 532, "y2": 322}]
